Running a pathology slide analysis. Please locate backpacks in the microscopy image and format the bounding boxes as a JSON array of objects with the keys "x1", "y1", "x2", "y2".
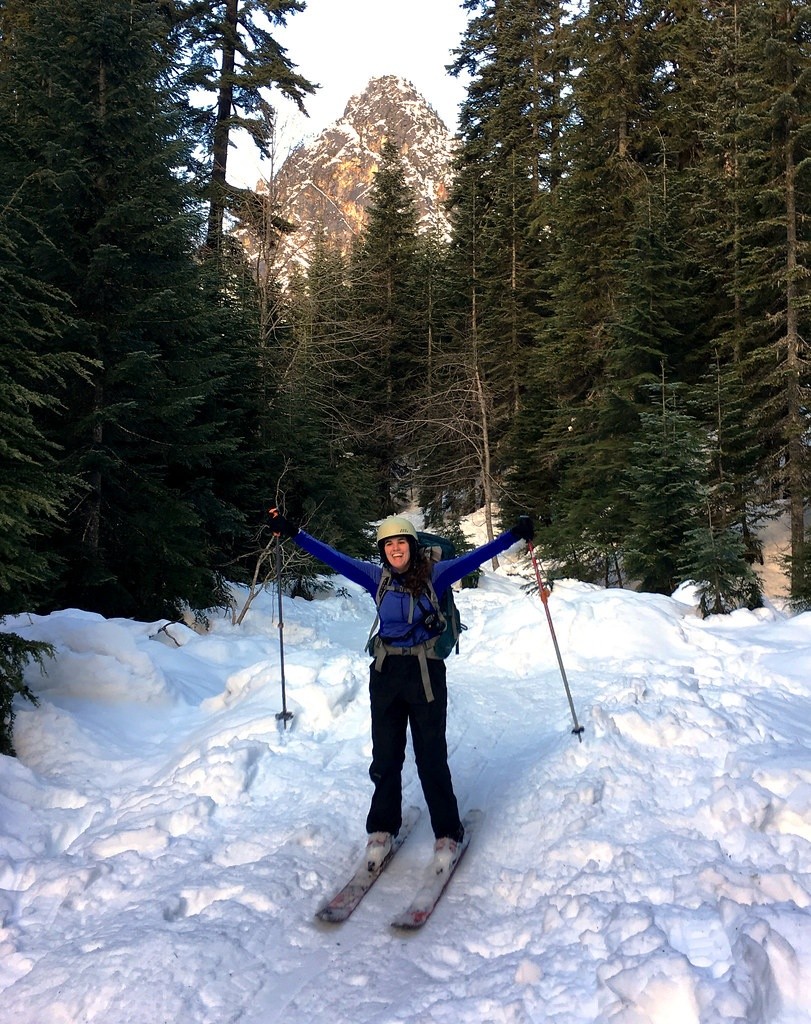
[{"x1": 364, "y1": 531, "x2": 468, "y2": 658}]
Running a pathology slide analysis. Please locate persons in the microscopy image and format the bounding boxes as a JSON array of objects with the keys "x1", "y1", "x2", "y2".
[{"x1": 269, "y1": 508, "x2": 538, "y2": 872}]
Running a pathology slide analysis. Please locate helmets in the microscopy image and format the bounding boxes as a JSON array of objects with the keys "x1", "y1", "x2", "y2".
[{"x1": 377, "y1": 517, "x2": 418, "y2": 545}]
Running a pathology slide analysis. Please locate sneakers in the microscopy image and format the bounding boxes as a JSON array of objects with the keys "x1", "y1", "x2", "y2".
[
  {"x1": 433, "y1": 835, "x2": 460, "y2": 863},
  {"x1": 364, "y1": 832, "x2": 392, "y2": 868}
]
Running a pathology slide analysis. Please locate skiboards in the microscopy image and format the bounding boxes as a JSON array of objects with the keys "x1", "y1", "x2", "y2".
[{"x1": 313, "y1": 806, "x2": 486, "y2": 932}]
